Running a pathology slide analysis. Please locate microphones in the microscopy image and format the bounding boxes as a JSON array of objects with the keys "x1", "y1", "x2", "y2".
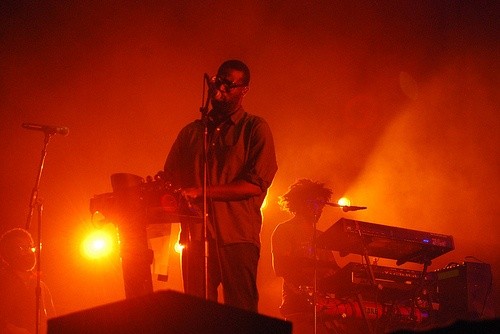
[
  {"x1": 205, "y1": 73, "x2": 221, "y2": 100},
  {"x1": 22, "y1": 123, "x2": 69, "y2": 136},
  {"x1": 343, "y1": 206, "x2": 367, "y2": 212}
]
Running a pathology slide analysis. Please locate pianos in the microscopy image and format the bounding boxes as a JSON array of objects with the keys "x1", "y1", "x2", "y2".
[
  {"x1": 316, "y1": 217, "x2": 454, "y2": 265},
  {"x1": 334, "y1": 261, "x2": 433, "y2": 299}
]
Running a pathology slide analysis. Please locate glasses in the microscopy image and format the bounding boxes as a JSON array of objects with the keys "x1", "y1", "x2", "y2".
[{"x1": 211, "y1": 75, "x2": 247, "y2": 88}]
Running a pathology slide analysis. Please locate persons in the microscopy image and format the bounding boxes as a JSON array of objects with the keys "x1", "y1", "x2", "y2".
[
  {"x1": 1, "y1": 228, "x2": 61, "y2": 333},
  {"x1": 162, "y1": 60, "x2": 279, "y2": 310},
  {"x1": 272, "y1": 181, "x2": 338, "y2": 301}
]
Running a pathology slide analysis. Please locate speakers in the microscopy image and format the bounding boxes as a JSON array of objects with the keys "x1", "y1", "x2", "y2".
[{"x1": 46, "y1": 289, "x2": 291, "y2": 334}]
